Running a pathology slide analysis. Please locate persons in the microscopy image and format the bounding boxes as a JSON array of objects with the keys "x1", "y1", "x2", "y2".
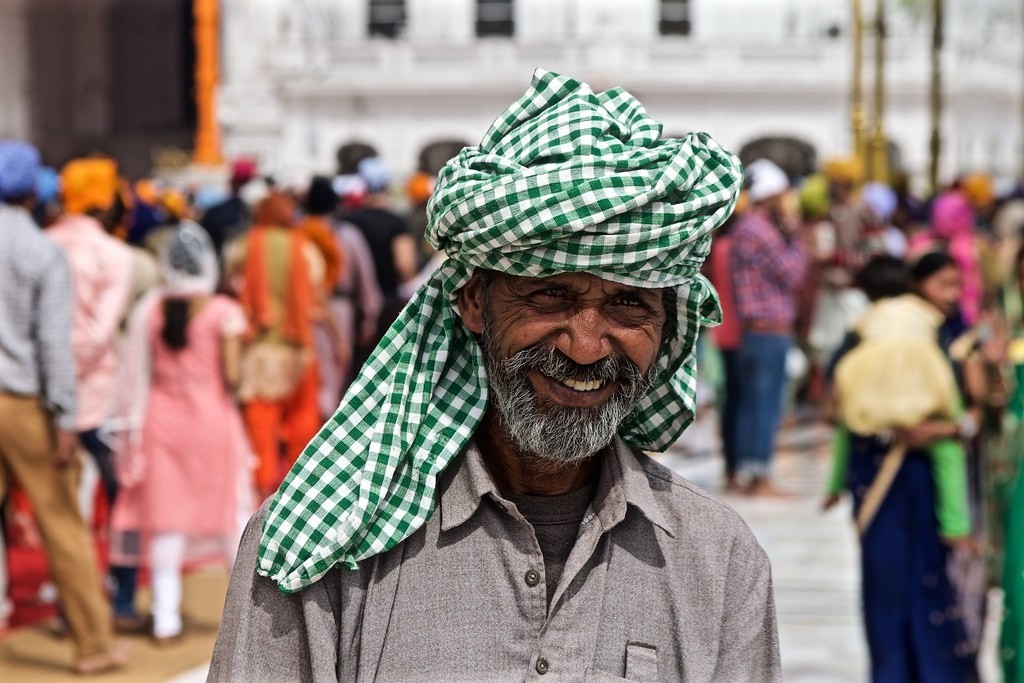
[
  {"x1": 709, "y1": 158, "x2": 1024, "y2": 683},
  {"x1": 205, "y1": 69, "x2": 782, "y2": 683},
  {"x1": 0, "y1": 117, "x2": 436, "y2": 671}
]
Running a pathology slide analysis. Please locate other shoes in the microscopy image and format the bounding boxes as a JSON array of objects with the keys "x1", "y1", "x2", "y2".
[
  {"x1": 111, "y1": 611, "x2": 151, "y2": 633},
  {"x1": 74, "y1": 641, "x2": 132, "y2": 673}
]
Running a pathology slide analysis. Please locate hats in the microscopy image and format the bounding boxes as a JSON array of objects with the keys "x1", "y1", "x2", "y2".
[
  {"x1": 747, "y1": 158, "x2": 789, "y2": 203},
  {"x1": 0, "y1": 138, "x2": 57, "y2": 202},
  {"x1": 60, "y1": 158, "x2": 117, "y2": 213}
]
